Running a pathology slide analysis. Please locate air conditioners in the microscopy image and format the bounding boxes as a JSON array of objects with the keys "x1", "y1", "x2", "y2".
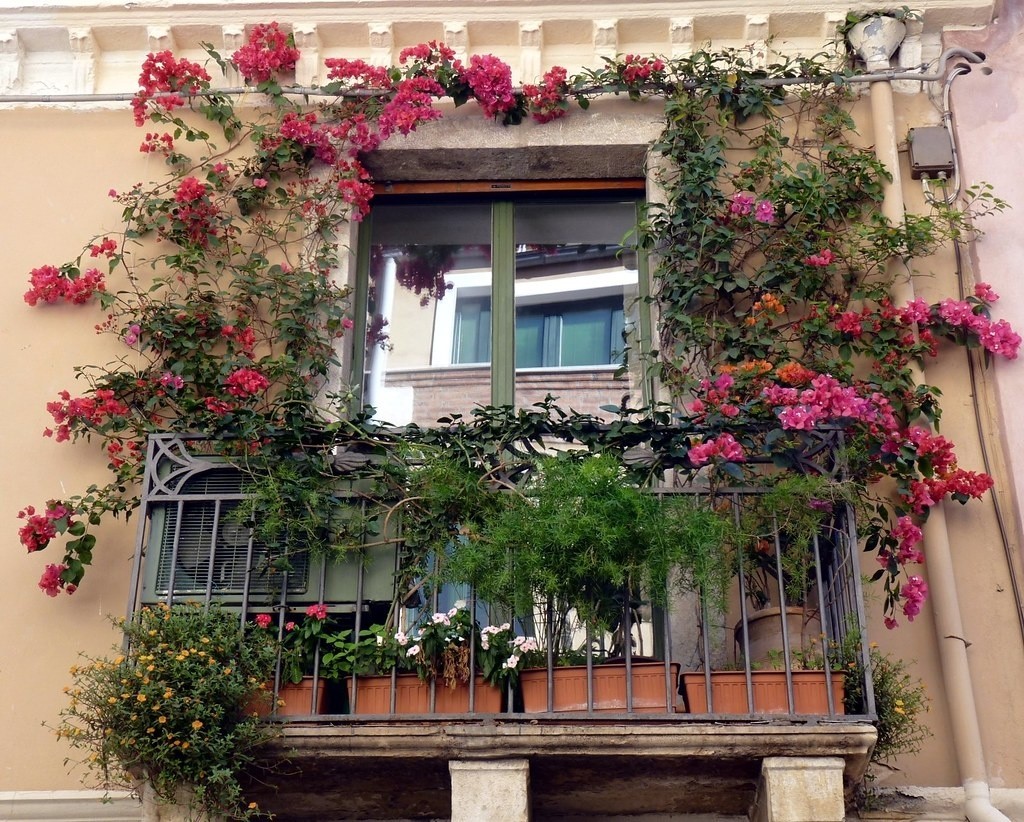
[{"x1": 136, "y1": 451, "x2": 407, "y2": 616}]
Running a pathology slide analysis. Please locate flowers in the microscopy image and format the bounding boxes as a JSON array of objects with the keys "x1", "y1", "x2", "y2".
[
  {"x1": 57, "y1": 595, "x2": 285, "y2": 816},
  {"x1": 794, "y1": 625, "x2": 949, "y2": 750},
  {"x1": 8, "y1": 19, "x2": 668, "y2": 608},
  {"x1": 239, "y1": 594, "x2": 543, "y2": 670},
  {"x1": 671, "y1": 161, "x2": 1022, "y2": 630}
]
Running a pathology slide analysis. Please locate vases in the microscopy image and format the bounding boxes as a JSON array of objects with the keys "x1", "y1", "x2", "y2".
[
  {"x1": 344, "y1": 671, "x2": 506, "y2": 726},
  {"x1": 520, "y1": 660, "x2": 682, "y2": 723},
  {"x1": 678, "y1": 669, "x2": 848, "y2": 723},
  {"x1": 733, "y1": 605, "x2": 832, "y2": 672},
  {"x1": 235, "y1": 673, "x2": 330, "y2": 727}
]
